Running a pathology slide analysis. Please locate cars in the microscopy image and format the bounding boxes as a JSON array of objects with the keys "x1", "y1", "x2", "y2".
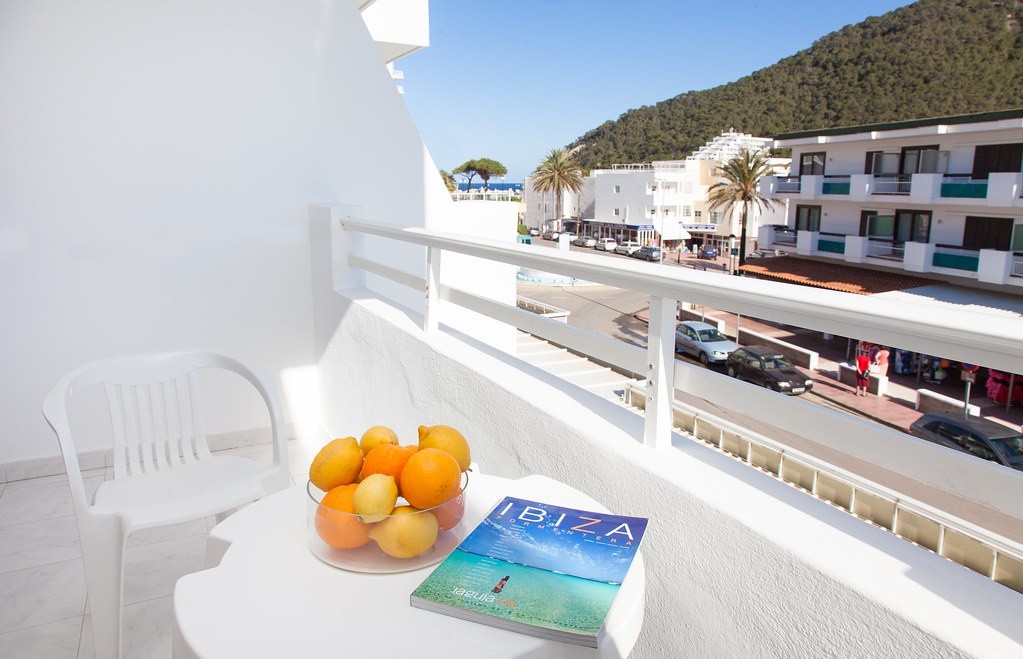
[
  {"x1": 573, "y1": 236, "x2": 596, "y2": 248},
  {"x1": 558, "y1": 232, "x2": 578, "y2": 244},
  {"x1": 909, "y1": 411, "x2": 1023, "y2": 473},
  {"x1": 543, "y1": 229, "x2": 560, "y2": 241},
  {"x1": 676, "y1": 320, "x2": 736, "y2": 365},
  {"x1": 531, "y1": 228, "x2": 540, "y2": 237},
  {"x1": 697, "y1": 244, "x2": 717, "y2": 261},
  {"x1": 632, "y1": 246, "x2": 666, "y2": 261},
  {"x1": 614, "y1": 242, "x2": 641, "y2": 256},
  {"x1": 594, "y1": 238, "x2": 616, "y2": 251},
  {"x1": 727, "y1": 345, "x2": 813, "y2": 396}
]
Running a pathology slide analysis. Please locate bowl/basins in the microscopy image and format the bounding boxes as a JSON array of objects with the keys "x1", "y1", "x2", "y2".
[{"x1": 306, "y1": 463, "x2": 467, "y2": 577}]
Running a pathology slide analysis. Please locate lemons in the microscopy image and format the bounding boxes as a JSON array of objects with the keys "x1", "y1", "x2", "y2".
[
  {"x1": 368, "y1": 506, "x2": 439, "y2": 558},
  {"x1": 309, "y1": 436, "x2": 363, "y2": 492},
  {"x1": 418, "y1": 424, "x2": 472, "y2": 473},
  {"x1": 354, "y1": 473, "x2": 398, "y2": 524},
  {"x1": 359, "y1": 425, "x2": 399, "y2": 455}
]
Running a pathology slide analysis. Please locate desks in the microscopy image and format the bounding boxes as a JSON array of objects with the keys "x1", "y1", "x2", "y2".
[{"x1": 173, "y1": 459, "x2": 648, "y2": 659}]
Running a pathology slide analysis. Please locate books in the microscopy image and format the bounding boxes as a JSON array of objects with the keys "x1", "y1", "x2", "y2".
[{"x1": 410, "y1": 494, "x2": 651, "y2": 648}]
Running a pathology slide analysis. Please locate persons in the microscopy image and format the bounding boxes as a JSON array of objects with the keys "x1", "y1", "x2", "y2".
[{"x1": 853, "y1": 350, "x2": 871, "y2": 397}]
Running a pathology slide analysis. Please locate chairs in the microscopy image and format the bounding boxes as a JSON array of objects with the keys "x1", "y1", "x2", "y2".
[{"x1": 39, "y1": 348, "x2": 296, "y2": 659}]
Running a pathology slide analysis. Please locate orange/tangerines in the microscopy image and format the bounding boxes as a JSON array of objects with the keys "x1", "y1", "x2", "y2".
[
  {"x1": 314, "y1": 483, "x2": 373, "y2": 550},
  {"x1": 361, "y1": 445, "x2": 464, "y2": 532}
]
